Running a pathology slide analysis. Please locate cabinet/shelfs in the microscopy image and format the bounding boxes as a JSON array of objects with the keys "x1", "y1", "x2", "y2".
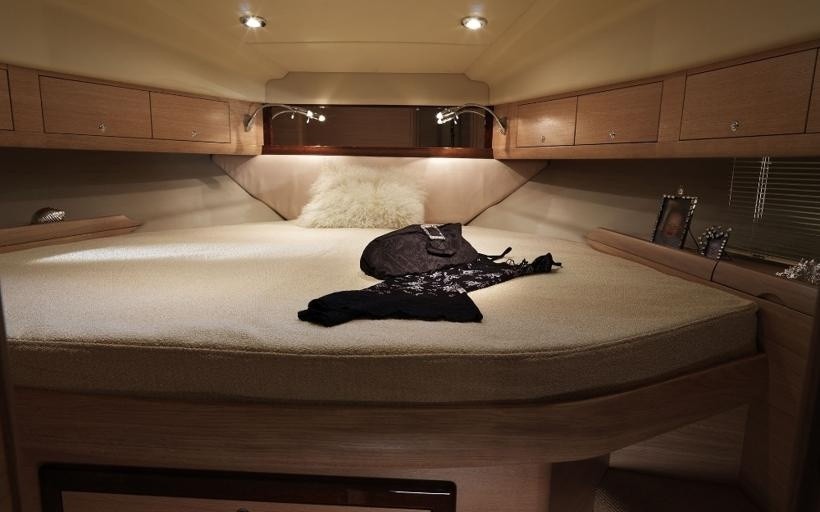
[
  {"x1": 2, "y1": 61, "x2": 263, "y2": 155},
  {"x1": 588, "y1": 223, "x2": 819, "y2": 510},
  {"x1": 491, "y1": 39, "x2": 820, "y2": 161}
]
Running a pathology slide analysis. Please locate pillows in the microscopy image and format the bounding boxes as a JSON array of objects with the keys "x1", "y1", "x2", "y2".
[{"x1": 297, "y1": 160, "x2": 427, "y2": 229}]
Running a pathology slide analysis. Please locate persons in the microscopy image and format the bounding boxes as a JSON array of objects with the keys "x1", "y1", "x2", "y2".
[{"x1": 656, "y1": 209, "x2": 686, "y2": 247}]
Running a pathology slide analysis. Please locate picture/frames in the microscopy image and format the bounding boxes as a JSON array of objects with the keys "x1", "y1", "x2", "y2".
[{"x1": 652, "y1": 184, "x2": 732, "y2": 261}]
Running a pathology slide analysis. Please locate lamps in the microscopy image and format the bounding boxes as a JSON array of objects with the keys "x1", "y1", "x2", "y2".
[
  {"x1": 435, "y1": 102, "x2": 507, "y2": 135},
  {"x1": 244, "y1": 104, "x2": 326, "y2": 131}
]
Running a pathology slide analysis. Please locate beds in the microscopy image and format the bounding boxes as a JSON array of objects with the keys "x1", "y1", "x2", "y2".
[{"x1": 1, "y1": 155, "x2": 763, "y2": 510}]
